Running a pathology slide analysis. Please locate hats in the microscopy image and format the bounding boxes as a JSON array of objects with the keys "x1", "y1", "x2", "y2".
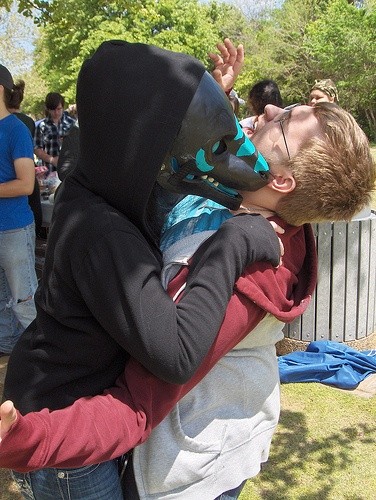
[
  {"x1": 310, "y1": 78, "x2": 340, "y2": 102},
  {"x1": 0, "y1": 64, "x2": 14, "y2": 91}
]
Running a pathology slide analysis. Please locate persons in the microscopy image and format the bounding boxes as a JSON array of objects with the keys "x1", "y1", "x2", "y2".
[
  {"x1": 3, "y1": 39, "x2": 285, "y2": 500},
  {"x1": 0, "y1": 34, "x2": 375, "y2": 500},
  {"x1": 0, "y1": 75, "x2": 341, "y2": 227},
  {"x1": 0, "y1": 63, "x2": 40, "y2": 353}
]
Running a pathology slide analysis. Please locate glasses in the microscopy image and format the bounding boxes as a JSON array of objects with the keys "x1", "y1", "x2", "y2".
[{"x1": 274, "y1": 102, "x2": 299, "y2": 165}]
